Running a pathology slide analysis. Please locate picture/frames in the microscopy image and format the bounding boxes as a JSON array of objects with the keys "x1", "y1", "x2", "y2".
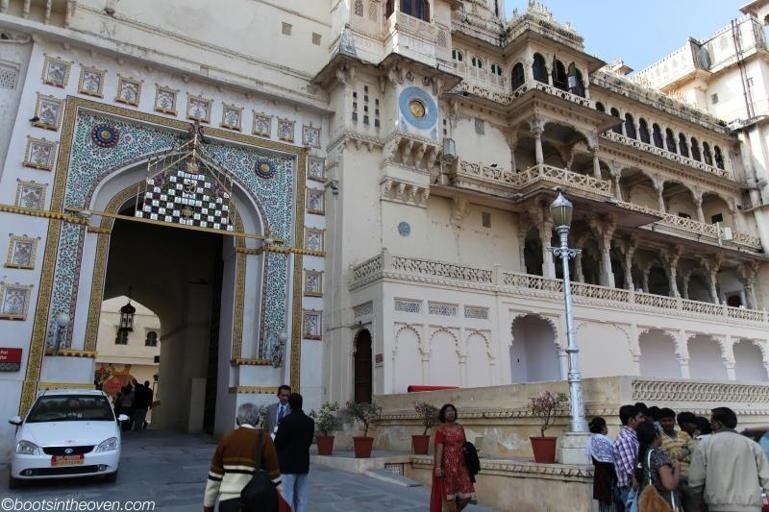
[
  {"x1": 301, "y1": 155, "x2": 325, "y2": 340},
  {"x1": 0, "y1": 54, "x2": 73, "y2": 321},
  {"x1": 77, "y1": 63, "x2": 322, "y2": 150}
]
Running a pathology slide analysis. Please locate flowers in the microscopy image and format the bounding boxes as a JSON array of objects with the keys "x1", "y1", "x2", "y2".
[
  {"x1": 414, "y1": 402, "x2": 439, "y2": 435},
  {"x1": 309, "y1": 401, "x2": 339, "y2": 435},
  {"x1": 527, "y1": 389, "x2": 569, "y2": 437},
  {"x1": 346, "y1": 399, "x2": 382, "y2": 436}
]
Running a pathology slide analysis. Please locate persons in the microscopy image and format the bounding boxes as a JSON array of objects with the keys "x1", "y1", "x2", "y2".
[
  {"x1": 588, "y1": 402, "x2": 769, "y2": 511},
  {"x1": 113, "y1": 378, "x2": 153, "y2": 432},
  {"x1": 430, "y1": 403, "x2": 478, "y2": 512},
  {"x1": 203, "y1": 404, "x2": 284, "y2": 512},
  {"x1": 274, "y1": 392, "x2": 314, "y2": 512},
  {"x1": 263, "y1": 385, "x2": 293, "y2": 444}
]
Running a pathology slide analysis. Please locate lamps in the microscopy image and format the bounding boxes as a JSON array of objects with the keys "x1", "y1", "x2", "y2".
[
  {"x1": 54, "y1": 313, "x2": 71, "y2": 350},
  {"x1": 121, "y1": 286, "x2": 134, "y2": 333},
  {"x1": 442, "y1": 137, "x2": 456, "y2": 162},
  {"x1": 270, "y1": 332, "x2": 288, "y2": 368}
]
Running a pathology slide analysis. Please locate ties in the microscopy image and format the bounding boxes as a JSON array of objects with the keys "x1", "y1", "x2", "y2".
[{"x1": 277, "y1": 407, "x2": 284, "y2": 424}]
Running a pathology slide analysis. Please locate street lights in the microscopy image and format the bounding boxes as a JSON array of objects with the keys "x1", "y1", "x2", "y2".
[{"x1": 546, "y1": 192, "x2": 589, "y2": 434}]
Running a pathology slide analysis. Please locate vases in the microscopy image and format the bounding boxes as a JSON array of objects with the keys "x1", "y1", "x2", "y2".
[
  {"x1": 413, "y1": 435, "x2": 430, "y2": 454},
  {"x1": 353, "y1": 436, "x2": 374, "y2": 459},
  {"x1": 316, "y1": 435, "x2": 335, "y2": 455},
  {"x1": 530, "y1": 437, "x2": 556, "y2": 463}
]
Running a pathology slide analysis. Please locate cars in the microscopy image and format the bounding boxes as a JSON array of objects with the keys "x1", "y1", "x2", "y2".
[{"x1": 9, "y1": 387, "x2": 129, "y2": 489}]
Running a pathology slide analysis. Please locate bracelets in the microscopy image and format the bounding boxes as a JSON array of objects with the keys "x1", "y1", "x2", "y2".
[{"x1": 434, "y1": 467, "x2": 440, "y2": 469}]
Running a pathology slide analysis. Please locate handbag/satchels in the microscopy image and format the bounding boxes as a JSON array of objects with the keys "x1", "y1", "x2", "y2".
[
  {"x1": 637, "y1": 484, "x2": 672, "y2": 511},
  {"x1": 241, "y1": 470, "x2": 280, "y2": 511}
]
